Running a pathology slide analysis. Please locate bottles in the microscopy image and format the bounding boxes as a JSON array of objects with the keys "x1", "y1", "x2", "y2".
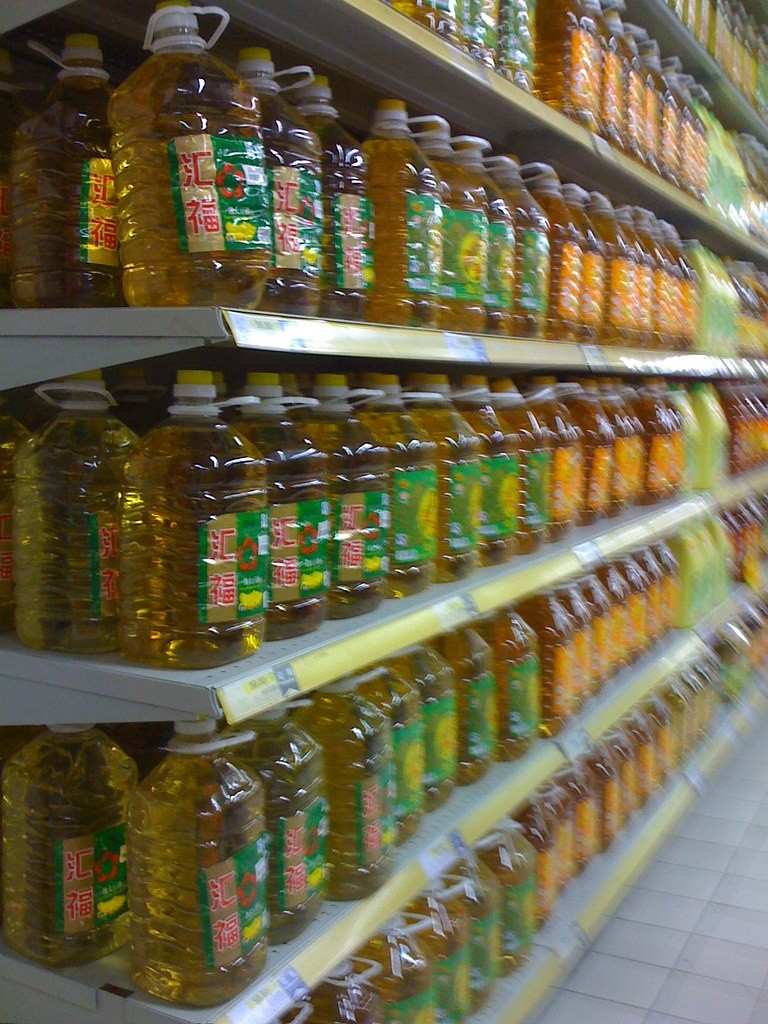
[{"x1": 0, "y1": 0, "x2": 768, "y2": 1024}]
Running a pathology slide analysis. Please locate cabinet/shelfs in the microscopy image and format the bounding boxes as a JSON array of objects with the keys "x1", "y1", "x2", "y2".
[{"x1": 0, "y1": 0, "x2": 767, "y2": 1024}]
